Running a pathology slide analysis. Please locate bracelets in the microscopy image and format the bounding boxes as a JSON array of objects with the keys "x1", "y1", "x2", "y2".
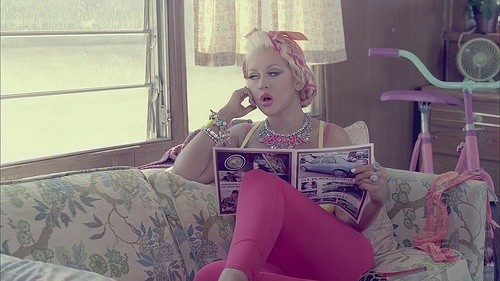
[{"x1": 201, "y1": 110, "x2": 231, "y2": 145}]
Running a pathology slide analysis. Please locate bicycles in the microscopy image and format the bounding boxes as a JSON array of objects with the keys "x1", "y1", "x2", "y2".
[{"x1": 366, "y1": 47, "x2": 500, "y2": 281}]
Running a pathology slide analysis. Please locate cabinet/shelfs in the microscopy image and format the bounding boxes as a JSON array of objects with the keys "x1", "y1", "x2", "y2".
[{"x1": 415, "y1": 85, "x2": 500, "y2": 201}]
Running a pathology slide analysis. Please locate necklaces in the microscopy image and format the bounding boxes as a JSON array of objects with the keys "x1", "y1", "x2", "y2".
[{"x1": 258, "y1": 113, "x2": 312, "y2": 149}]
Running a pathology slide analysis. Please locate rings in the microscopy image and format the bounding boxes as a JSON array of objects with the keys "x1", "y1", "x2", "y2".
[
  {"x1": 374, "y1": 162, "x2": 380, "y2": 172},
  {"x1": 370, "y1": 175, "x2": 378, "y2": 184}
]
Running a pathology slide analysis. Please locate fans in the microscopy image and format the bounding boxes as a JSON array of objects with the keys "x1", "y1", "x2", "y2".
[{"x1": 456, "y1": 37, "x2": 500, "y2": 83}]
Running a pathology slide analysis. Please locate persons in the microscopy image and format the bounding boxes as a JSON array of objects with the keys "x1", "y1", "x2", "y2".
[
  {"x1": 222, "y1": 172, "x2": 237, "y2": 182},
  {"x1": 173, "y1": 31, "x2": 388, "y2": 281},
  {"x1": 221, "y1": 190, "x2": 239, "y2": 213}
]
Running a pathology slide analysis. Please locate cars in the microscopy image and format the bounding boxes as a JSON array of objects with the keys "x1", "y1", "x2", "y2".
[
  {"x1": 299, "y1": 155, "x2": 368, "y2": 178},
  {"x1": 321, "y1": 182, "x2": 354, "y2": 193}
]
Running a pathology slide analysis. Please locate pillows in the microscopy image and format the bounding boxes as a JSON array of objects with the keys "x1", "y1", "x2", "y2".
[{"x1": 334, "y1": 121, "x2": 397, "y2": 258}]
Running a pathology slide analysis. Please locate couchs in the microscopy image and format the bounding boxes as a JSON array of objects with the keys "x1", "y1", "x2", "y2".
[{"x1": 0, "y1": 163, "x2": 500, "y2": 281}]
